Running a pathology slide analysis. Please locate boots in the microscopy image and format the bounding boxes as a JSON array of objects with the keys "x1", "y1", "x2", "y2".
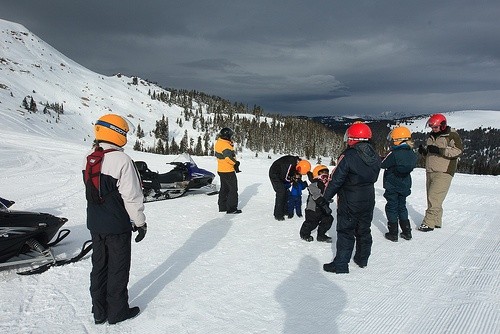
[
  {"x1": 399, "y1": 221, "x2": 412, "y2": 240},
  {"x1": 385, "y1": 223, "x2": 399, "y2": 242}
]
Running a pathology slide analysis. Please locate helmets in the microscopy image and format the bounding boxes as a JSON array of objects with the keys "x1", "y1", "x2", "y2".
[
  {"x1": 220, "y1": 128, "x2": 235, "y2": 139},
  {"x1": 390, "y1": 127, "x2": 412, "y2": 139},
  {"x1": 428, "y1": 114, "x2": 446, "y2": 130},
  {"x1": 95, "y1": 114, "x2": 129, "y2": 146},
  {"x1": 296, "y1": 160, "x2": 311, "y2": 175},
  {"x1": 312, "y1": 165, "x2": 329, "y2": 177},
  {"x1": 346, "y1": 123, "x2": 372, "y2": 138}
]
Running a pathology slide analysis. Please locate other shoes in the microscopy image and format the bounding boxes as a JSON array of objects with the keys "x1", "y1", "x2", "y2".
[
  {"x1": 318, "y1": 236, "x2": 332, "y2": 241},
  {"x1": 109, "y1": 307, "x2": 140, "y2": 324},
  {"x1": 296, "y1": 213, "x2": 303, "y2": 218},
  {"x1": 353, "y1": 256, "x2": 367, "y2": 268},
  {"x1": 323, "y1": 263, "x2": 350, "y2": 273},
  {"x1": 219, "y1": 210, "x2": 242, "y2": 214},
  {"x1": 418, "y1": 224, "x2": 441, "y2": 231},
  {"x1": 95, "y1": 317, "x2": 108, "y2": 324},
  {"x1": 288, "y1": 213, "x2": 294, "y2": 218},
  {"x1": 301, "y1": 236, "x2": 313, "y2": 242},
  {"x1": 275, "y1": 217, "x2": 285, "y2": 221}
]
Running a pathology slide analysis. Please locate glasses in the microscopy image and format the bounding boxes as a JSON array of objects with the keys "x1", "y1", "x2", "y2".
[{"x1": 318, "y1": 174, "x2": 328, "y2": 179}]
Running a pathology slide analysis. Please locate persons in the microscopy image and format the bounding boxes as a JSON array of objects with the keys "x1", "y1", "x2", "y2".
[
  {"x1": 323, "y1": 123, "x2": 381, "y2": 273},
  {"x1": 82, "y1": 115, "x2": 147, "y2": 324},
  {"x1": 381, "y1": 127, "x2": 417, "y2": 242},
  {"x1": 269, "y1": 156, "x2": 311, "y2": 221},
  {"x1": 300, "y1": 166, "x2": 334, "y2": 243},
  {"x1": 284, "y1": 173, "x2": 306, "y2": 218},
  {"x1": 418, "y1": 114, "x2": 463, "y2": 231},
  {"x1": 215, "y1": 127, "x2": 242, "y2": 214}
]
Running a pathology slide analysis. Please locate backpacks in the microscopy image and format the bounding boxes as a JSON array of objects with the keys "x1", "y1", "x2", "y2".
[{"x1": 83, "y1": 146, "x2": 120, "y2": 204}]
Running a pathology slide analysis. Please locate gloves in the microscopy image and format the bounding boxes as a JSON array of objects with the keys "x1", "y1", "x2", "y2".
[
  {"x1": 418, "y1": 145, "x2": 427, "y2": 156},
  {"x1": 427, "y1": 145, "x2": 439, "y2": 155},
  {"x1": 320, "y1": 200, "x2": 332, "y2": 215},
  {"x1": 131, "y1": 222, "x2": 147, "y2": 242},
  {"x1": 235, "y1": 161, "x2": 241, "y2": 173}
]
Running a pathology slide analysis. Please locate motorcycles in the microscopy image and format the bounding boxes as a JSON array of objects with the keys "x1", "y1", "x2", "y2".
[
  {"x1": 0, "y1": 197, "x2": 92, "y2": 276},
  {"x1": 134, "y1": 151, "x2": 220, "y2": 202}
]
